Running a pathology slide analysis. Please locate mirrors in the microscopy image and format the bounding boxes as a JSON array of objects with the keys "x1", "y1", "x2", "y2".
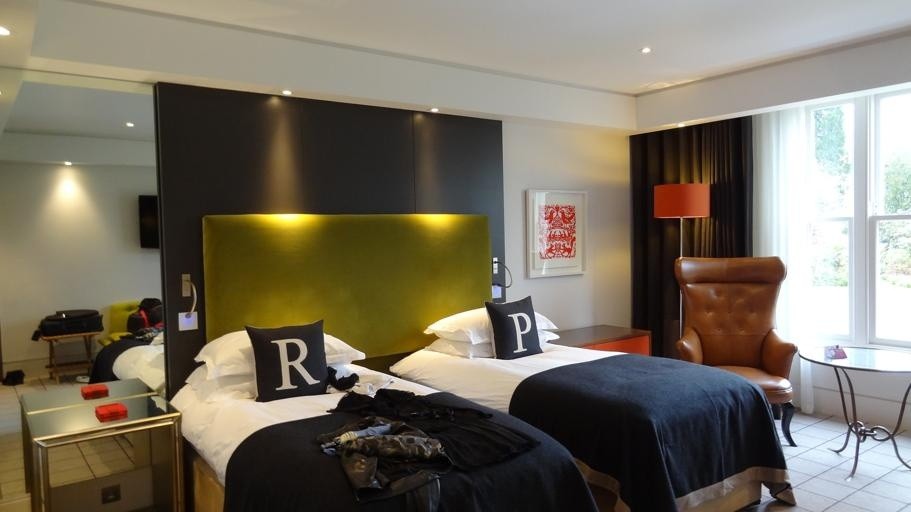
[{"x1": 0, "y1": 65, "x2": 169, "y2": 511}]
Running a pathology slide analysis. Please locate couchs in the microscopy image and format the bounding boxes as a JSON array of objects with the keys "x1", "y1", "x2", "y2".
[{"x1": 674, "y1": 256, "x2": 797, "y2": 448}]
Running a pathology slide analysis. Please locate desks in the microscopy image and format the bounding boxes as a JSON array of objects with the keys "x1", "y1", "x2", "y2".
[
  {"x1": 798, "y1": 348, "x2": 911, "y2": 482},
  {"x1": 41, "y1": 331, "x2": 102, "y2": 381}
]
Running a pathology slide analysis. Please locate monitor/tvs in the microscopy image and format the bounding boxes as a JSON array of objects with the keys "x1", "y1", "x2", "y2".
[{"x1": 138, "y1": 195, "x2": 159, "y2": 249}]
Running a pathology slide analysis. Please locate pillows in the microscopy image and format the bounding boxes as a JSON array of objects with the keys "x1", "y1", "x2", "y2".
[
  {"x1": 185, "y1": 364, "x2": 355, "y2": 404},
  {"x1": 244, "y1": 319, "x2": 331, "y2": 402},
  {"x1": 485, "y1": 295, "x2": 544, "y2": 360},
  {"x1": 425, "y1": 331, "x2": 560, "y2": 360},
  {"x1": 423, "y1": 306, "x2": 559, "y2": 345},
  {"x1": 194, "y1": 330, "x2": 366, "y2": 380}
]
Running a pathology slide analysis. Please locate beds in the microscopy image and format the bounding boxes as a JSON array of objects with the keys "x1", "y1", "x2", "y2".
[
  {"x1": 389, "y1": 343, "x2": 762, "y2": 512},
  {"x1": 98, "y1": 301, "x2": 166, "y2": 397},
  {"x1": 172, "y1": 365, "x2": 599, "y2": 512}
]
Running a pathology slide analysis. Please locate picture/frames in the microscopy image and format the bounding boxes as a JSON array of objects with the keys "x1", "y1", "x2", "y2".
[{"x1": 526, "y1": 189, "x2": 588, "y2": 279}]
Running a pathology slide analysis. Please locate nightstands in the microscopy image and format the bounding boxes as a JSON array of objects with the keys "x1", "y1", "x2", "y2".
[
  {"x1": 547, "y1": 325, "x2": 653, "y2": 356},
  {"x1": 19, "y1": 377, "x2": 158, "y2": 493},
  {"x1": 25, "y1": 395, "x2": 183, "y2": 512}
]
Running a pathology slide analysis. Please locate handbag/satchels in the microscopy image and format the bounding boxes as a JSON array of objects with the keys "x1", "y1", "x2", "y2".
[{"x1": 31, "y1": 309, "x2": 104, "y2": 341}]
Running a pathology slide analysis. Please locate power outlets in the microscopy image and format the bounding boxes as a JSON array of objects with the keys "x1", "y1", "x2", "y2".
[{"x1": 102, "y1": 485, "x2": 121, "y2": 504}]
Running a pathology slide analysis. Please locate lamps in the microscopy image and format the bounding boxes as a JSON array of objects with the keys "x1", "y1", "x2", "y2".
[
  {"x1": 654, "y1": 182, "x2": 710, "y2": 337},
  {"x1": 492, "y1": 258, "x2": 513, "y2": 299},
  {"x1": 178, "y1": 274, "x2": 199, "y2": 332}
]
